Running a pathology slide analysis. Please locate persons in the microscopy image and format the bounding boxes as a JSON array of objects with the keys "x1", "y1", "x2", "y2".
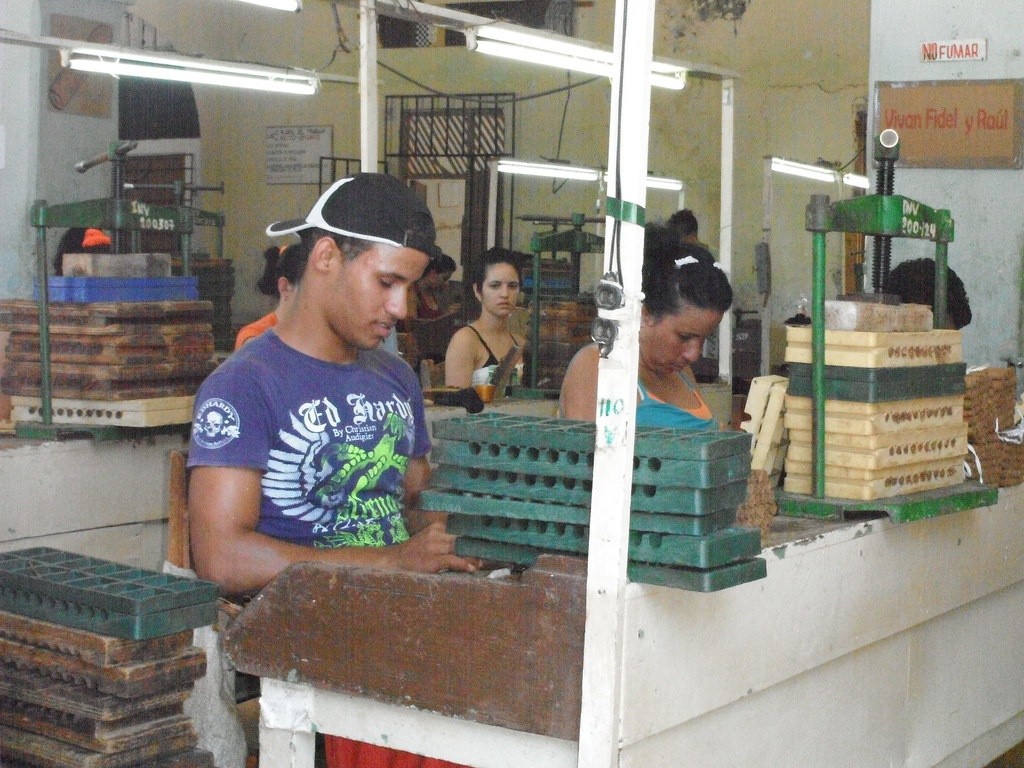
[
  {"x1": 554, "y1": 222, "x2": 732, "y2": 430},
  {"x1": 445, "y1": 247, "x2": 530, "y2": 401},
  {"x1": 667, "y1": 211, "x2": 722, "y2": 265},
  {"x1": 884, "y1": 259, "x2": 972, "y2": 332},
  {"x1": 188, "y1": 173, "x2": 476, "y2": 596},
  {"x1": 234, "y1": 243, "x2": 309, "y2": 350}
]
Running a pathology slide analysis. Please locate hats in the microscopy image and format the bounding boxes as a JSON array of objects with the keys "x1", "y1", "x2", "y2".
[
  {"x1": 58, "y1": 227, "x2": 114, "y2": 255},
  {"x1": 266, "y1": 172, "x2": 440, "y2": 260}
]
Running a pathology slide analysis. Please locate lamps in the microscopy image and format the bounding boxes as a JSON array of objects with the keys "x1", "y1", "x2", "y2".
[
  {"x1": 62, "y1": 46, "x2": 321, "y2": 96},
  {"x1": 240, "y1": 0, "x2": 303, "y2": 13},
  {"x1": 762, "y1": 154, "x2": 871, "y2": 190},
  {"x1": 464, "y1": 24, "x2": 690, "y2": 91},
  {"x1": 487, "y1": 157, "x2": 684, "y2": 193}
]
